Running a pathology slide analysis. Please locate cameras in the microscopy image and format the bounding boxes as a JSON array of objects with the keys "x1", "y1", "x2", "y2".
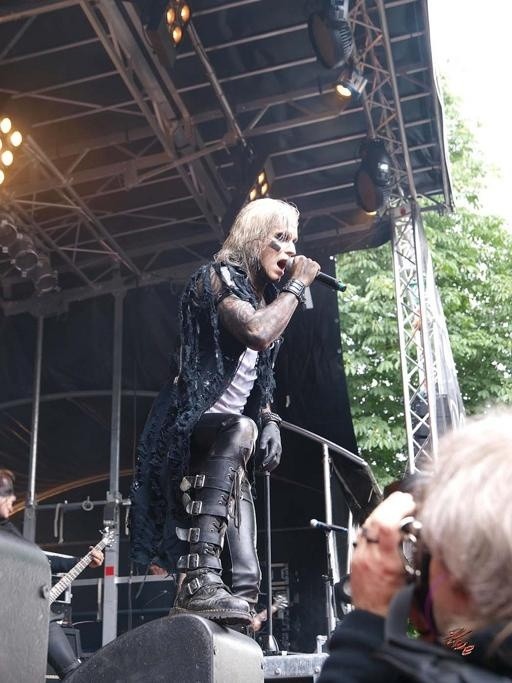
[{"x1": 382, "y1": 469, "x2": 429, "y2": 584}]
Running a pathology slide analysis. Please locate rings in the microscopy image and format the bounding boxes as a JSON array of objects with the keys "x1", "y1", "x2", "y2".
[{"x1": 366, "y1": 538, "x2": 380, "y2": 544}]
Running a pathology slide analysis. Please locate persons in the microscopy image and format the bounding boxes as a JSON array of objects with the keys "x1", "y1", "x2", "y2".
[
  {"x1": 128, "y1": 559, "x2": 178, "y2": 626},
  {"x1": 125, "y1": 197, "x2": 321, "y2": 635},
  {"x1": 315, "y1": 401, "x2": 512, "y2": 683},
  {"x1": 0, "y1": 468, "x2": 105, "y2": 681}
]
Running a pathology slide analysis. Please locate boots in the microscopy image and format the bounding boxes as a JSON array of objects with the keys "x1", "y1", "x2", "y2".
[{"x1": 167, "y1": 456, "x2": 254, "y2": 626}]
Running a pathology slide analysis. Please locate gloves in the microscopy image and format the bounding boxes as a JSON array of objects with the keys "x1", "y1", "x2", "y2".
[{"x1": 259, "y1": 421, "x2": 281, "y2": 473}]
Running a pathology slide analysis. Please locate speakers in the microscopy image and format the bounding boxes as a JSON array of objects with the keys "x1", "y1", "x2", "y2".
[
  {"x1": 412, "y1": 394, "x2": 454, "y2": 467},
  {"x1": 47, "y1": 626, "x2": 82, "y2": 675},
  {"x1": 263, "y1": 653, "x2": 330, "y2": 683},
  {"x1": 0, "y1": 526, "x2": 52, "y2": 683},
  {"x1": 59, "y1": 612, "x2": 263, "y2": 683}
]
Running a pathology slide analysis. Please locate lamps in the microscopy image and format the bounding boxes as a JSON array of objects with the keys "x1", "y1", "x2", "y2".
[
  {"x1": 332, "y1": 67, "x2": 368, "y2": 102},
  {"x1": 0, "y1": 113, "x2": 23, "y2": 185},
  {"x1": 146, "y1": 0, "x2": 191, "y2": 69},
  {"x1": 307, "y1": 0, "x2": 354, "y2": 70},
  {"x1": 353, "y1": 140, "x2": 391, "y2": 212},
  {"x1": 222, "y1": 169, "x2": 272, "y2": 240}
]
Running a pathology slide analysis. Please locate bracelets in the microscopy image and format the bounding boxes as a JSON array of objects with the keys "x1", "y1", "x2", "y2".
[{"x1": 279, "y1": 277, "x2": 307, "y2": 305}]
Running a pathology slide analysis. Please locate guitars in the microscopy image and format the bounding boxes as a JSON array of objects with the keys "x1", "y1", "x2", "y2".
[
  {"x1": 229, "y1": 595, "x2": 289, "y2": 637},
  {"x1": 51, "y1": 527, "x2": 115, "y2": 604}
]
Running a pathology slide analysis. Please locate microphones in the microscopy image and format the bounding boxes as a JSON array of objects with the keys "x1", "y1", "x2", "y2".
[
  {"x1": 285, "y1": 256, "x2": 347, "y2": 292},
  {"x1": 309, "y1": 519, "x2": 349, "y2": 533}
]
